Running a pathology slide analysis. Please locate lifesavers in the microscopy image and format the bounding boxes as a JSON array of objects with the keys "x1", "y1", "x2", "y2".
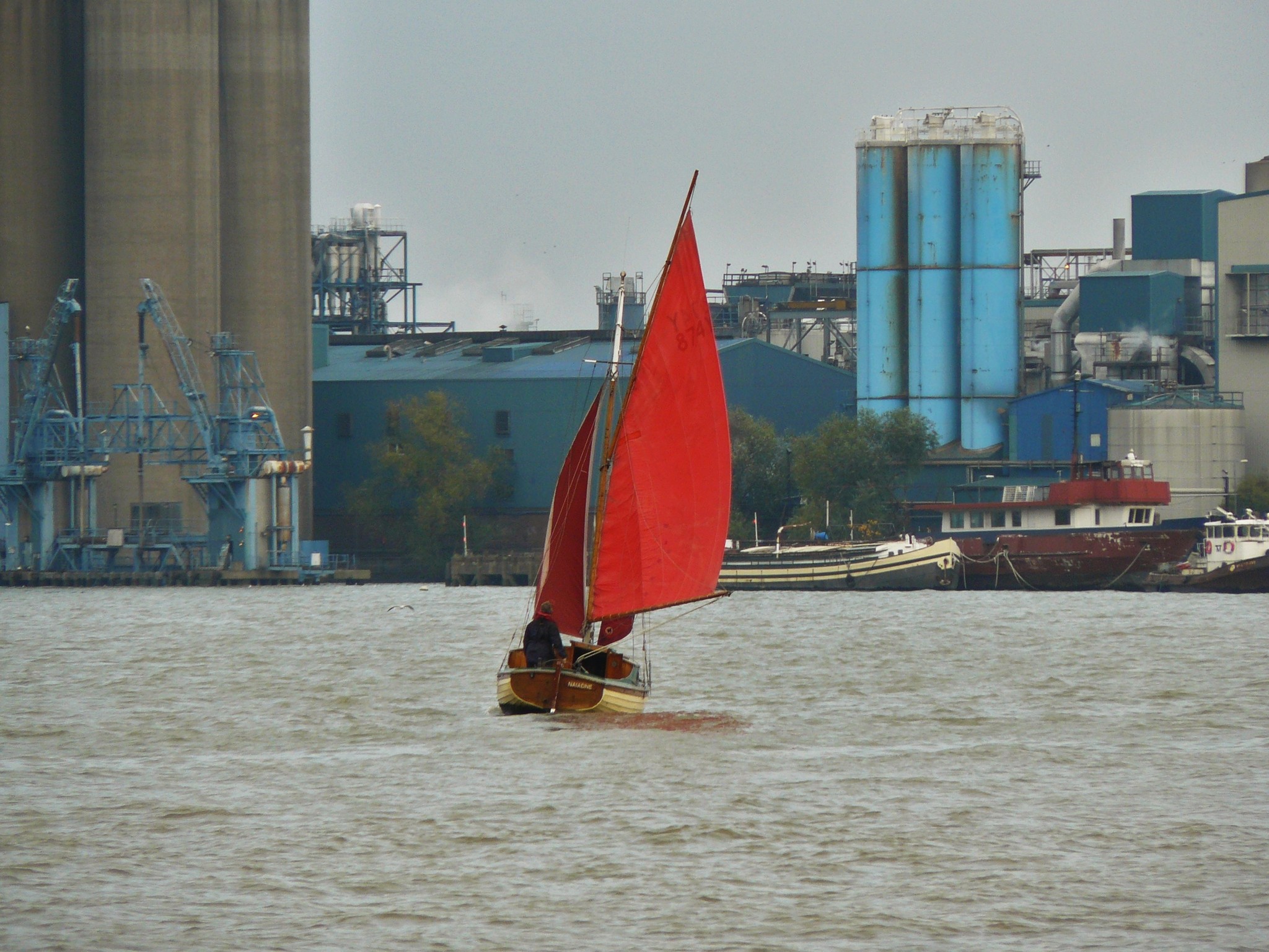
[
  {"x1": 1223, "y1": 541, "x2": 1234, "y2": 554},
  {"x1": 1205, "y1": 541, "x2": 1212, "y2": 554},
  {"x1": 845, "y1": 575, "x2": 856, "y2": 588}
]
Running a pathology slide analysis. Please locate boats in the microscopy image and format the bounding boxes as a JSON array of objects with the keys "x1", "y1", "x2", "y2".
[
  {"x1": 715, "y1": 502, "x2": 964, "y2": 592},
  {"x1": 910, "y1": 367, "x2": 1201, "y2": 593},
  {"x1": 1143, "y1": 504, "x2": 1269, "y2": 595}
]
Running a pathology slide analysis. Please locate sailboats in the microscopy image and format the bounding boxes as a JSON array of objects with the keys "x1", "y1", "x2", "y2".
[{"x1": 493, "y1": 168, "x2": 736, "y2": 719}]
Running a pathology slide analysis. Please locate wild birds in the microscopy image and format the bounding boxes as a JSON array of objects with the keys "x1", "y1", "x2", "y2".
[{"x1": 386, "y1": 605, "x2": 414, "y2": 612}]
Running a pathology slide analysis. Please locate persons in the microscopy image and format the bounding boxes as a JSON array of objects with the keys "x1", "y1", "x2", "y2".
[{"x1": 524, "y1": 601, "x2": 567, "y2": 670}]
